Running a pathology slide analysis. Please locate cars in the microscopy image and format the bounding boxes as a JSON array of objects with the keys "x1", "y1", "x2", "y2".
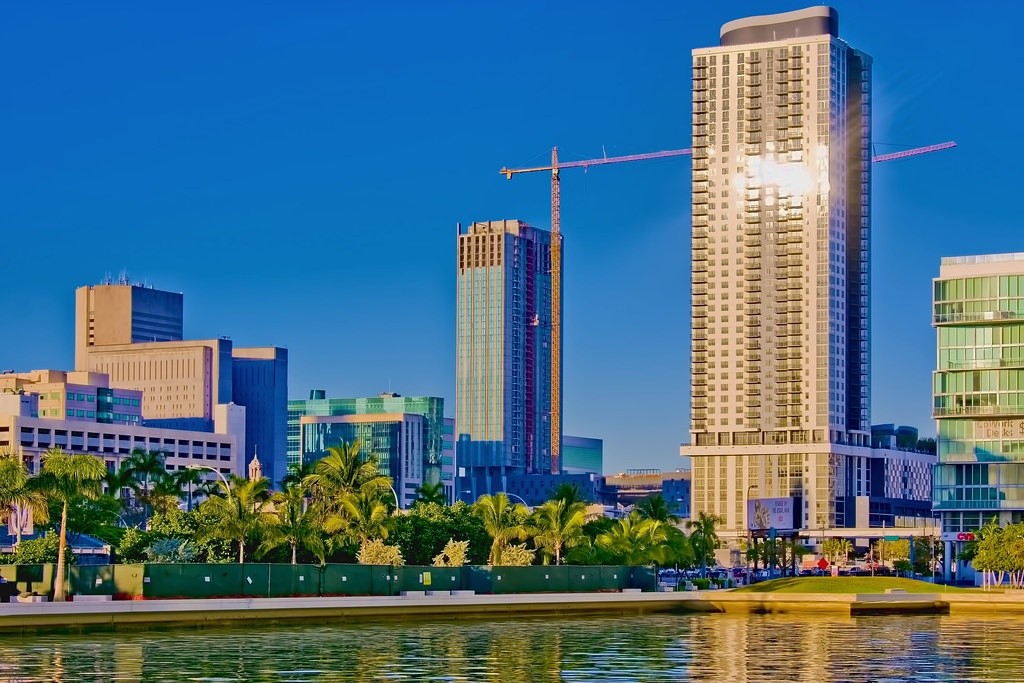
[
  {"x1": 756, "y1": 569, "x2": 799, "y2": 577},
  {"x1": 800, "y1": 567, "x2": 831, "y2": 577},
  {"x1": 658, "y1": 567, "x2": 754, "y2": 577},
  {"x1": 850, "y1": 567, "x2": 861, "y2": 572}
]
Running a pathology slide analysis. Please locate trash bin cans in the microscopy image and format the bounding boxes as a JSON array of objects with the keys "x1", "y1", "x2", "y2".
[{"x1": 723, "y1": 580, "x2": 728, "y2": 589}]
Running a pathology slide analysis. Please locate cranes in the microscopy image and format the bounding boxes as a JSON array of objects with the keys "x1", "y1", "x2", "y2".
[
  {"x1": 497, "y1": 146, "x2": 692, "y2": 471},
  {"x1": 871, "y1": 140, "x2": 960, "y2": 165}
]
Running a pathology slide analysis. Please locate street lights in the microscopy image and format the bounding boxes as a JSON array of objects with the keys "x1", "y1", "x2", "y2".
[
  {"x1": 456, "y1": 490, "x2": 472, "y2": 502},
  {"x1": 822, "y1": 520, "x2": 826, "y2": 577},
  {"x1": 184, "y1": 464, "x2": 233, "y2": 508},
  {"x1": 746, "y1": 485, "x2": 758, "y2": 584}
]
